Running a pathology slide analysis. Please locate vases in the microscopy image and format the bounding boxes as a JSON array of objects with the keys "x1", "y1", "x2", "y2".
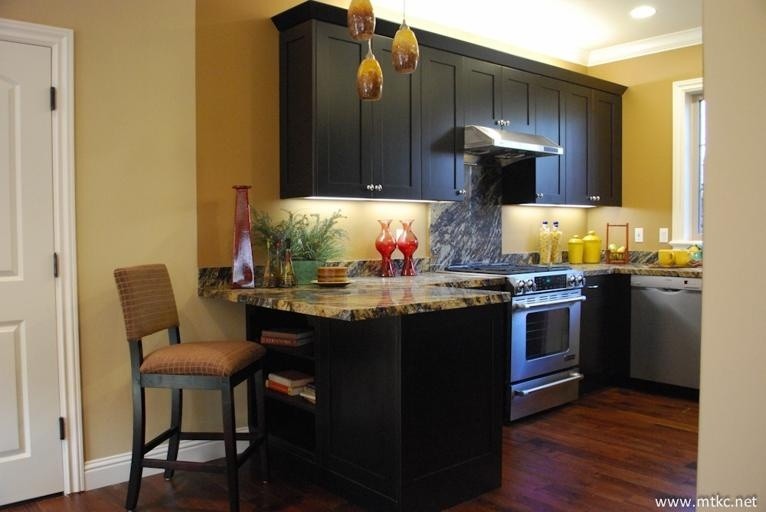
[{"x1": 373, "y1": 216, "x2": 419, "y2": 276}]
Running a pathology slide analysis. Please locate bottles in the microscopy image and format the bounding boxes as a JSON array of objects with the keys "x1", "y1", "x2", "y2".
[{"x1": 538, "y1": 217, "x2": 563, "y2": 264}]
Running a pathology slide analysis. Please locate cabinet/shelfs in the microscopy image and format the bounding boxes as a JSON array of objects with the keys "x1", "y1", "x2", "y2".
[
  {"x1": 423, "y1": 28, "x2": 496, "y2": 201},
  {"x1": 197, "y1": 258, "x2": 511, "y2": 511},
  {"x1": 565, "y1": 64, "x2": 630, "y2": 207},
  {"x1": 271, "y1": 0, "x2": 421, "y2": 200},
  {"x1": 501, "y1": 51, "x2": 567, "y2": 205},
  {"x1": 580, "y1": 249, "x2": 631, "y2": 401}
]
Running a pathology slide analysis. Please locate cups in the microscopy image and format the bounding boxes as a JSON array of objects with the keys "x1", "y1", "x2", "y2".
[{"x1": 657, "y1": 247, "x2": 692, "y2": 270}]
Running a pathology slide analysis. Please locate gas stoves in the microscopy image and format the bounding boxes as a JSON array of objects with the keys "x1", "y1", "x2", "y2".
[{"x1": 453, "y1": 260, "x2": 585, "y2": 293}]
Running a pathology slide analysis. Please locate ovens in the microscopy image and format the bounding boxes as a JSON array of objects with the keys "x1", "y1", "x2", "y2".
[{"x1": 511, "y1": 292, "x2": 584, "y2": 421}]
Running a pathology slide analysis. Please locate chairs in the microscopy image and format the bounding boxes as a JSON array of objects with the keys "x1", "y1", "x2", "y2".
[{"x1": 113, "y1": 263, "x2": 270, "y2": 512}]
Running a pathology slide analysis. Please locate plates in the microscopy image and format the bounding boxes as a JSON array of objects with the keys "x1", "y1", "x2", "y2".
[{"x1": 310, "y1": 279, "x2": 356, "y2": 288}]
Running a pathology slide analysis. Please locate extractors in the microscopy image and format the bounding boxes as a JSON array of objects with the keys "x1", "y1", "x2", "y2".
[{"x1": 460, "y1": 123, "x2": 564, "y2": 167}]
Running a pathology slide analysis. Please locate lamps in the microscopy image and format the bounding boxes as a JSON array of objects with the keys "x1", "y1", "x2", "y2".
[{"x1": 344, "y1": 0, "x2": 418, "y2": 102}]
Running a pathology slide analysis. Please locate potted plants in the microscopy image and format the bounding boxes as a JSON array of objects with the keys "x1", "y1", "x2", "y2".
[{"x1": 251, "y1": 206, "x2": 348, "y2": 281}]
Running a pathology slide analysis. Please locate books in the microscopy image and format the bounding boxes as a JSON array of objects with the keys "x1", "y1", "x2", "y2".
[
  {"x1": 259, "y1": 326, "x2": 314, "y2": 348},
  {"x1": 264, "y1": 371, "x2": 317, "y2": 401}
]
